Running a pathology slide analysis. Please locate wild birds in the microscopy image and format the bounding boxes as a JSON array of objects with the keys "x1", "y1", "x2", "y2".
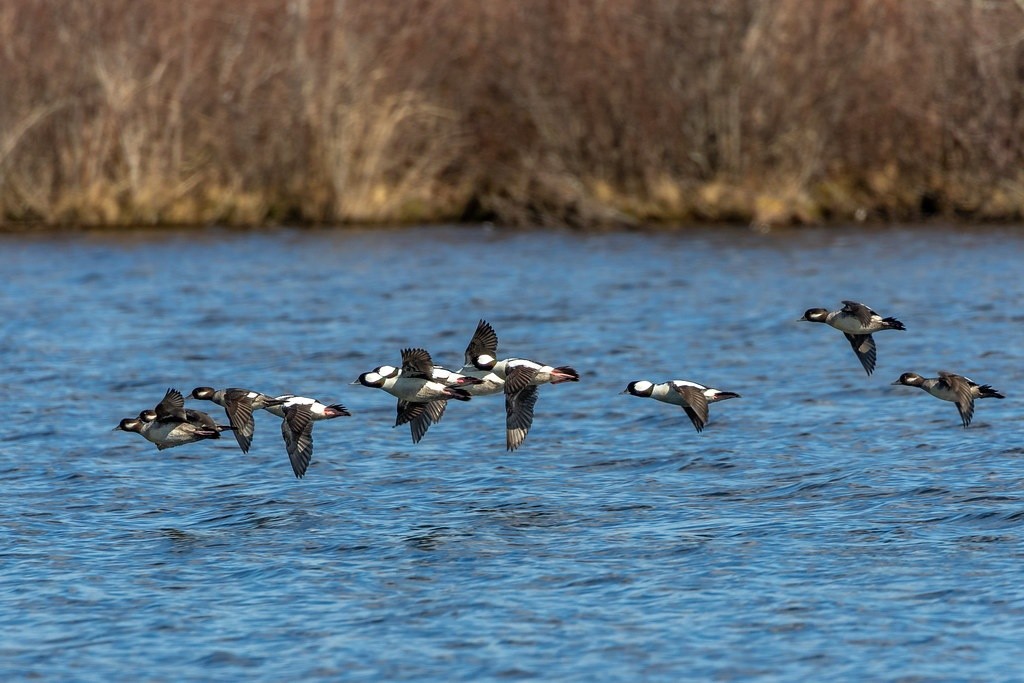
[
  {"x1": 620, "y1": 381, "x2": 741, "y2": 433},
  {"x1": 795, "y1": 300, "x2": 907, "y2": 376},
  {"x1": 259, "y1": 394, "x2": 351, "y2": 478},
  {"x1": 348, "y1": 349, "x2": 486, "y2": 445},
  {"x1": 449, "y1": 318, "x2": 580, "y2": 452},
  {"x1": 111, "y1": 388, "x2": 238, "y2": 451},
  {"x1": 890, "y1": 371, "x2": 1005, "y2": 427},
  {"x1": 186, "y1": 387, "x2": 290, "y2": 454}
]
